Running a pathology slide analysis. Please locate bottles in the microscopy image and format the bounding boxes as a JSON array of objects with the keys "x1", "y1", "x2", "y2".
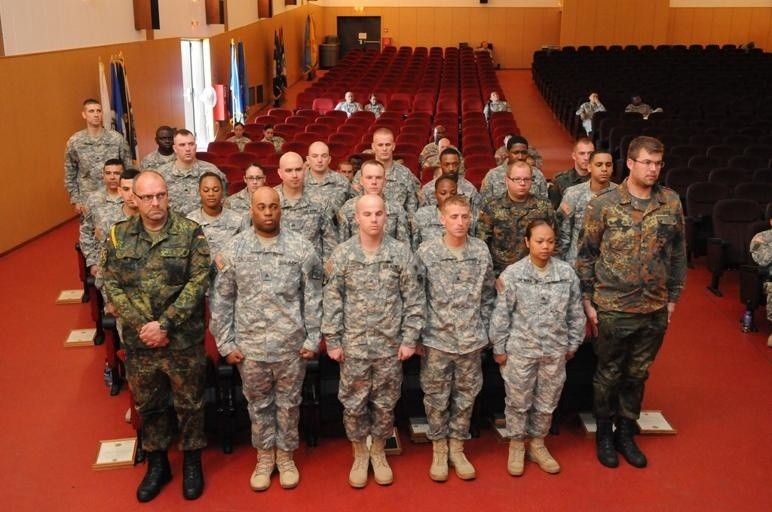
[{"x1": 741, "y1": 311, "x2": 752, "y2": 333}]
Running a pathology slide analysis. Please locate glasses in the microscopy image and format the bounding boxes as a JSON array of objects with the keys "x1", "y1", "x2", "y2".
[
  {"x1": 246, "y1": 176, "x2": 263, "y2": 182},
  {"x1": 510, "y1": 176, "x2": 532, "y2": 183},
  {"x1": 135, "y1": 193, "x2": 168, "y2": 201},
  {"x1": 635, "y1": 158, "x2": 665, "y2": 169}
]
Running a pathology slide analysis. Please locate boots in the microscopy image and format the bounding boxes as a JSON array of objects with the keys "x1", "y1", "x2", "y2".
[
  {"x1": 449, "y1": 438, "x2": 475, "y2": 480},
  {"x1": 527, "y1": 439, "x2": 560, "y2": 474},
  {"x1": 507, "y1": 439, "x2": 526, "y2": 475},
  {"x1": 429, "y1": 439, "x2": 448, "y2": 482},
  {"x1": 615, "y1": 419, "x2": 647, "y2": 468},
  {"x1": 137, "y1": 450, "x2": 173, "y2": 501},
  {"x1": 348, "y1": 439, "x2": 370, "y2": 488},
  {"x1": 182, "y1": 449, "x2": 203, "y2": 501},
  {"x1": 595, "y1": 422, "x2": 618, "y2": 467},
  {"x1": 371, "y1": 439, "x2": 393, "y2": 485},
  {"x1": 276, "y1": 449, "x2": 300, "y2": 489},
  {"x1": 250, "y1": 448, "x2": 276, "y2": 490}
]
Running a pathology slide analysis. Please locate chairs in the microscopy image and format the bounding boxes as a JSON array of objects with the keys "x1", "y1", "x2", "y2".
[
  {"x1": 187, "y1": 44, "x2": 596, "y2": 454},
  {"x1": 533, "y1": 40, "x2": 771, "y2": 335}
]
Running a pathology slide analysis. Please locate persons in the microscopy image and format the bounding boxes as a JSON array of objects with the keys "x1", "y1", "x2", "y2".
[
  {"x1": 99, "y1": 169, "x2": 214, "y2": 505},
  {"x1": 210, "y1": 184, "x2": 326, "y2": 493},
  {"x1": 364, "y1": 93, "x2": 386, "y2": 120},
  {"x1": 487, "y1": 218, "x2": 589, "y2": 478},
  {"x1": 576, "y1": 93, "x2": 607, "y2": 134},
  {"x1": 220, "y1": 164, "x2": 267, "y2": 225},
  {"x1": 140, "y1": 125, "x2": 177, "y2": 168},
  {"x1": 224, "y1": 123, "x2": 252, "y2": 152},
  {"x1": 749, "y1": 216, "x2": 771, "y2": 350},
  {"x1": 320, "y1": 192, "x2": 427, "y2": 489},
  {"x1": 185, "y1": 172, "x2": 250, "y2": 256},
  {"x1": 412, "y1": 194, "x2": 495, "y2": 484},
  {"x1": 624, "y1": 93, "x2": 653, "y2": 120},
  {"x1": 157, "y1": 128, "x2": 228, "y2": 218},
  {"x1": 64, "y1": 99, "x2": 141, "y2": 339},
  {"x1": 259, "y1": 124, "x2": 286, "y2": 152},
  {"x1": 333, "y1": 91, "x2": 364, "y2": 118},
  {"x1": 579, "y1": 134, "x2": 689, "y2": 470},
  {"x1": 483, "y1": 91, "x2": 512, "y2": 128},
  {"x1": 272, "y1": 126, "x2": 618, "y2": 280}
]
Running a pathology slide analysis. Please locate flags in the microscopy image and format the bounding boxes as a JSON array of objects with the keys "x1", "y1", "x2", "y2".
[
  {"x1": 303, "y1": 12, "x2": 317, "y2": 67},
  {"x1": 97, "y1": 51, "x2": 134, "y2": 162},
  {"x1": 228, "y1": 38, "x2": 240, "y2": 127},
  {"x1": 236, "y1": 40, "x2": 248, "y2": 121},
  {"x1": 270, "y1": 27, "x2": 285, "y2": 106}
]
